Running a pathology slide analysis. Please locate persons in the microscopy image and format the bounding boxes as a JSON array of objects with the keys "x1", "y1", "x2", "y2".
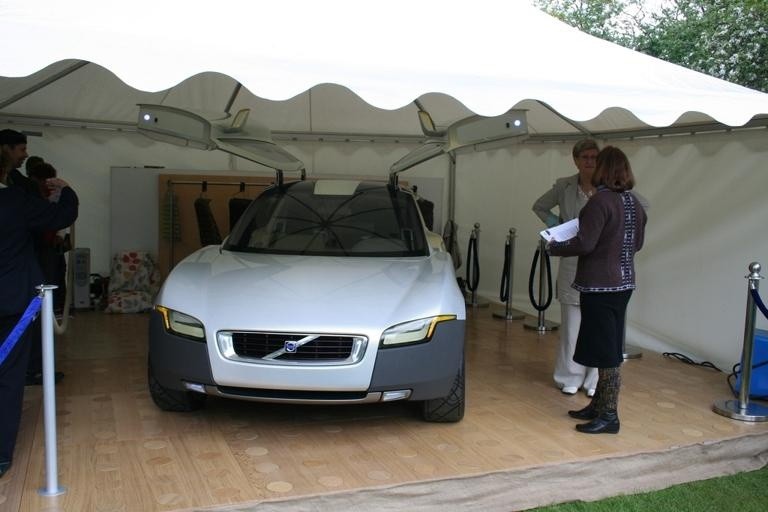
[
  {"x1": 0, "y1": 129, "x2": 79, "y2": 474},
  {"x1": 532, "y1": 139, "x2": 650, "y2": 398},
  {"x1": 545, "y1": 145, "x2": 647, "y2": 433}
]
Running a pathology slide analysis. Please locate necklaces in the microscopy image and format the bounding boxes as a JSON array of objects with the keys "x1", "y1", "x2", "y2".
[{"x1": 577, "y1": 183, "x2": 596, "y2": 201}]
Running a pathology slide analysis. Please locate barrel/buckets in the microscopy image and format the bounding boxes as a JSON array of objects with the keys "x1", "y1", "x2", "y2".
[{"x1": 734, "y1": 328, "x2": 768, "y2": 400}]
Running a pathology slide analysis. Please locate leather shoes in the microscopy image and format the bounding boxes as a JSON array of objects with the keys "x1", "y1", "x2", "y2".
[
  {"x1": 0, "y1": 461, "x2": 11, "y2": 477},
  {"x1": 24, "y1": 370, "x2": 64, "y2": 386},
  {"x1": 562, "y1": 387, "x2": 595, "y2": 397}
]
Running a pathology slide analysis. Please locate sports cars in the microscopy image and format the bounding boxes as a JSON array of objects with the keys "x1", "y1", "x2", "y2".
[{"x1": 145, "y1": 177, "x2": 469, "y2": 425}]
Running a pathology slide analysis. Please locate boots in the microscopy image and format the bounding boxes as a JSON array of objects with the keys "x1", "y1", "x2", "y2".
[
  {"x1": 568, "y1": 403, "x2": 599, "y2": 420},
  {"x1": 576, "y1": 409, "x2": 619, "y2": 433}
]
